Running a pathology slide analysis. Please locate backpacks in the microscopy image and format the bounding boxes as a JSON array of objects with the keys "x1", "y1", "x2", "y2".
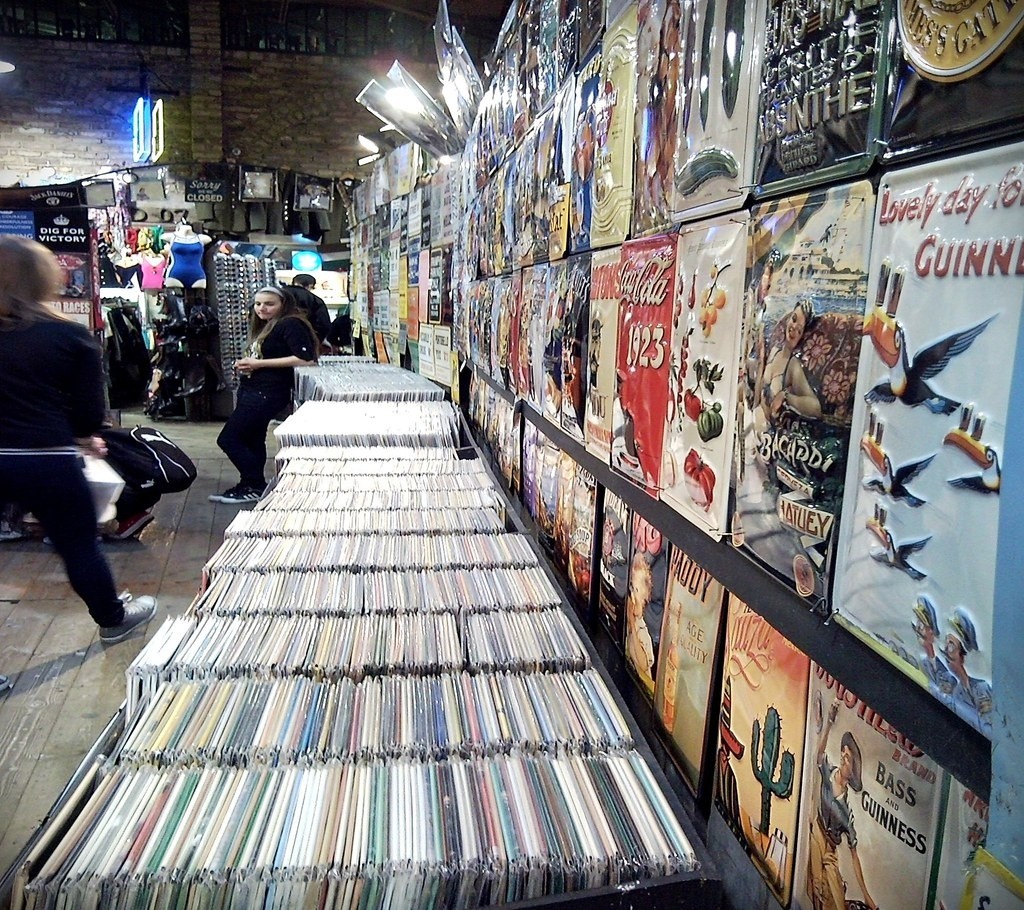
[{"x1": 100, "y1": 425, "x2": 198, "y2": 494}]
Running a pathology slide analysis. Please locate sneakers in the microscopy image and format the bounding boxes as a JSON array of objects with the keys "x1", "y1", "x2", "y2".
[
  {"x1": 114, "y1": 510, "x2": 154, "y2": 538},
  {"x1": 208, "y1": 480, "x2": 268, "y2": 504},
  {"x1": 0, "y1": 675, "x2": 9, "y2": 692},
  {"x1": 99, "y1": 589, "x2": 157, "y2": 643}
]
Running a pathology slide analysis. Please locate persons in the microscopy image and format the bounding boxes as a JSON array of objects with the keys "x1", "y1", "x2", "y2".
[
  {"x1": 283, "y1": 274, "x2": 331, "y2": 345},
  {"x1": 96, "y1": 424, "x2": 160, "y2": 541},
  {"x1": 138, "y1": 188, "x2": 149, "y2": 201},
  {"x1": 208, "y1": 285, "x2": 318, "y2": 504},
  {"x1": 114, "y1": 225, "x2": 212, "y2": 294},
  {"x1": 0, "y1": 236, "x2": 157, "y2": 644}
]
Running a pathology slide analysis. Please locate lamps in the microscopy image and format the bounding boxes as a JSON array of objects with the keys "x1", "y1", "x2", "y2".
[{"x1": 358, "y1": 131, "x2": 395, "y2": 154}]
